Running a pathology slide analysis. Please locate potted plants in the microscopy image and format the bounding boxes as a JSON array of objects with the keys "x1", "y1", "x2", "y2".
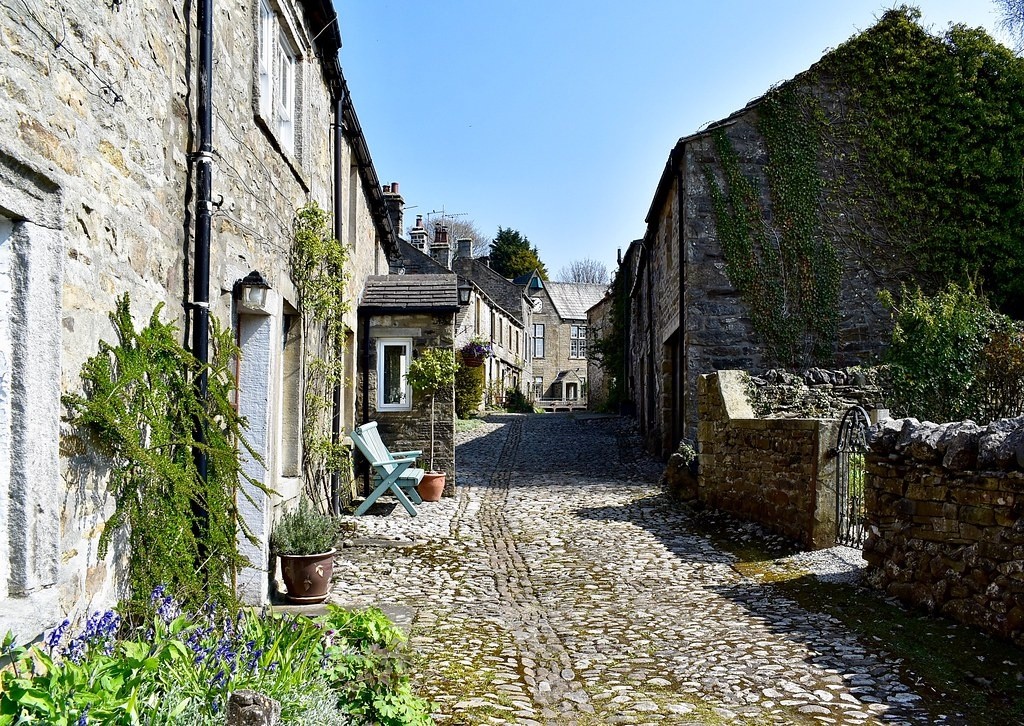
[
  {"x1": 401, "y1": 347, "x2": 461, "y2": 502},
  {"x1": 270, "y1": 493, "x2": 341, "y2": 597}
]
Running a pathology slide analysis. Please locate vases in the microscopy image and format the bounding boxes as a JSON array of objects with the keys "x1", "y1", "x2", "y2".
[{"x1": 464, "y1": 357, "x2": 484, "y2": 367}]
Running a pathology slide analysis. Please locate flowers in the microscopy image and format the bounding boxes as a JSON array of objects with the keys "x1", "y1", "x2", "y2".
[{"x1": 460, "y1": 341, "x2": 495, "y2": 358}]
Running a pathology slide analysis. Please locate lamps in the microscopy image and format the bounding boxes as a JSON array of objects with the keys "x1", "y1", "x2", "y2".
[
  {"x1": 457, "y1": 279, "x2": 474, "y2": 306},
  {"x1": 221, "y1": 269, "x2": 272, "y2": 310}
]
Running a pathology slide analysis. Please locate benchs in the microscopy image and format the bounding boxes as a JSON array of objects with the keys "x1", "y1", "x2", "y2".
[{"x1": 349, "y1": 420, "x2": 425, "y2": 517}]
